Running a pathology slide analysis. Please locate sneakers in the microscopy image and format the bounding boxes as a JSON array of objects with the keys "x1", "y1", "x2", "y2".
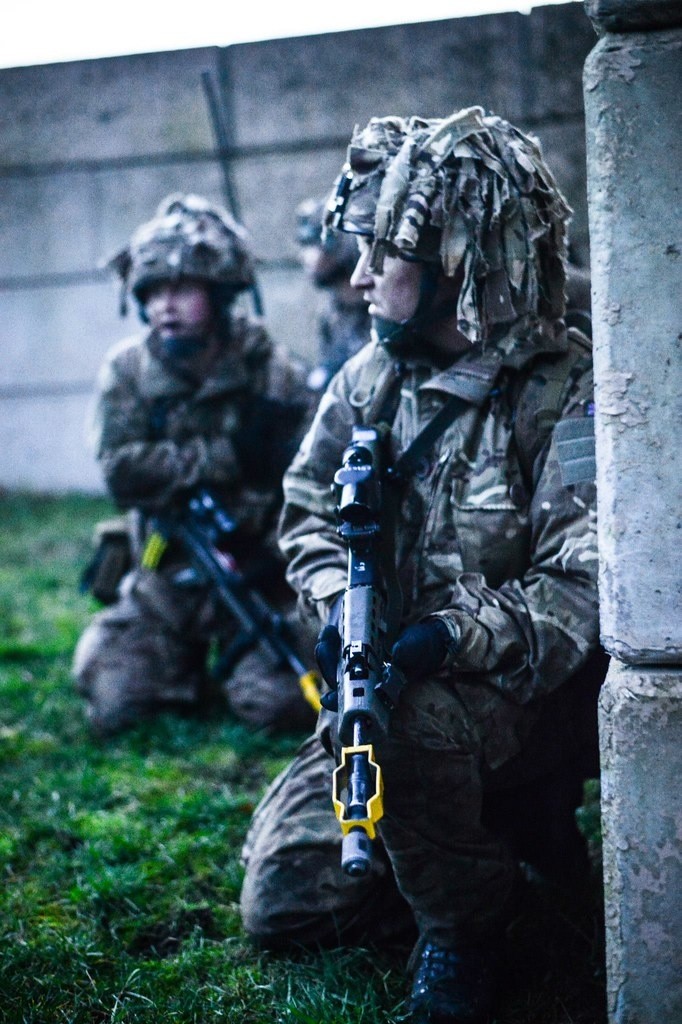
[{"x1": 408, "y1": 932, "x2": 507, "y2": 1024}]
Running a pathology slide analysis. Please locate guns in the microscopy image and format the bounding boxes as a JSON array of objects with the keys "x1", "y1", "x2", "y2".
[
  {"x1": 140, "y1": 477, "x2": 329, "y2": 713},
  {"x1": 330, "y1": 417, "x2": 410, "y2": 879}
]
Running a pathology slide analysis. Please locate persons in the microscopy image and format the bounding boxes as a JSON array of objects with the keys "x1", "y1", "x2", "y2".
[
  {"x1": 240, "y1": 106, "x2": 604, "y2": 1024},
  {"x1": 72, "y1": 192, "x2": 324, "y2": 729},
  {"x1": 296, "y1": 195, "x2": 372, "y2": 389}
]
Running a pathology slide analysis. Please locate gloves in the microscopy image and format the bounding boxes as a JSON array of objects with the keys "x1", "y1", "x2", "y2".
[{"x1": 315, "y1": 615, "x2": 451, "y2": 715}]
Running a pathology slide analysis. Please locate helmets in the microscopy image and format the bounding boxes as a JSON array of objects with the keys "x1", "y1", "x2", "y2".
[
  {"x1": 297, "y1": 194, "x2": 361, "y2": 246},
  {"x1": 321, "y1": 112, "x2": 570, "y2": 270},
  {"x1": 132, "y1": 196, "x2": 253, "y2": 301}
]
